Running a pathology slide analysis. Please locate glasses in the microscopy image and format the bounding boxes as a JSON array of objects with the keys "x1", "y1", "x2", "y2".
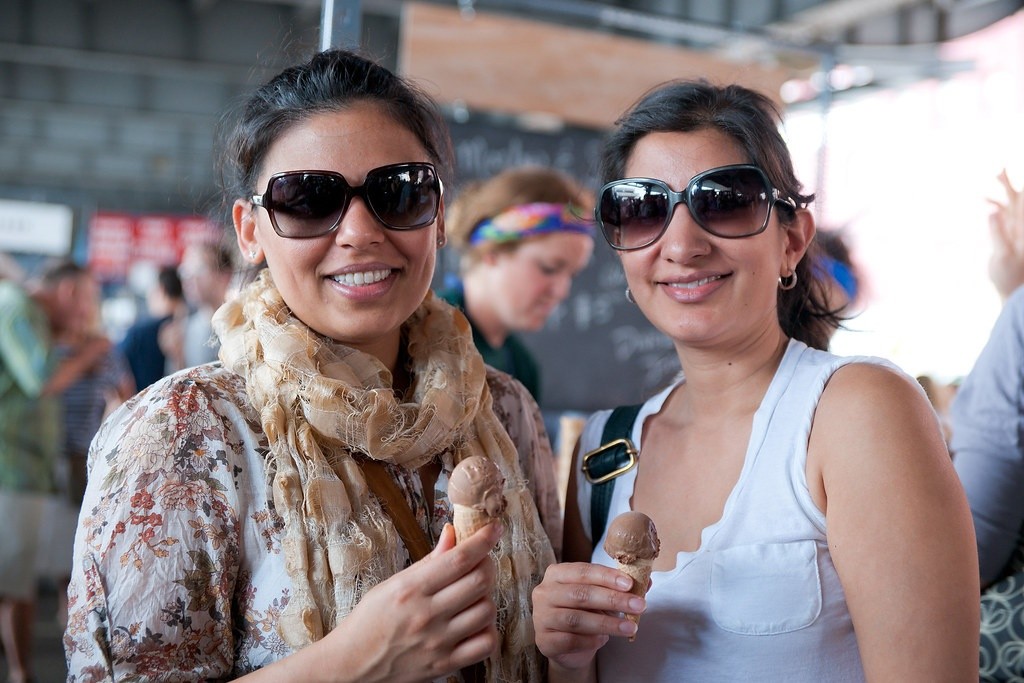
[
  {"x1": 593, "y1": 163, "x2": 796, "y2": 251},
  {"x1": 249, "y1": 161, "x2": 444, "y2": 239}
]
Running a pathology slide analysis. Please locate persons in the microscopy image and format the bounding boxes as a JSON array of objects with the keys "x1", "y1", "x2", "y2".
[
  {"x1": 530, "y1": 78, "x2": 980, "y2": 683},
  {"x1": 2, "y1": 162, "x2": 1024, "y2": 682},
  {"x1": 62, "y1": 48, "x2": 565, "y2": 683}
]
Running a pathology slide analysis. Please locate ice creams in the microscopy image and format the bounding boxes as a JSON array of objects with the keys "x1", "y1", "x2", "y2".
[
  {"x1": 604, "y1": 511, "x2": 661, "y2": 640},
  {"x1": 448, "y1": 456, "x2": 504, "y2": 542}
]
